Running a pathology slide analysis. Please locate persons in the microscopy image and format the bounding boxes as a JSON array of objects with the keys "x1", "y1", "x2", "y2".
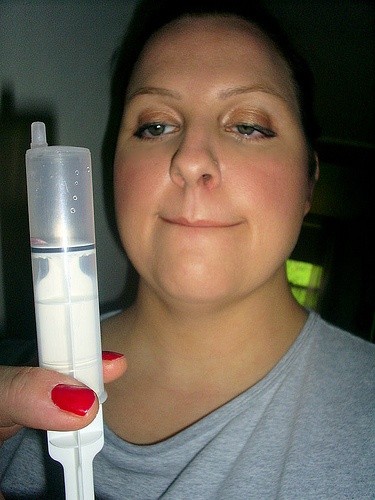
[{"x1": 1, "y1": 0, "x2": 375, "y2": 500}]
